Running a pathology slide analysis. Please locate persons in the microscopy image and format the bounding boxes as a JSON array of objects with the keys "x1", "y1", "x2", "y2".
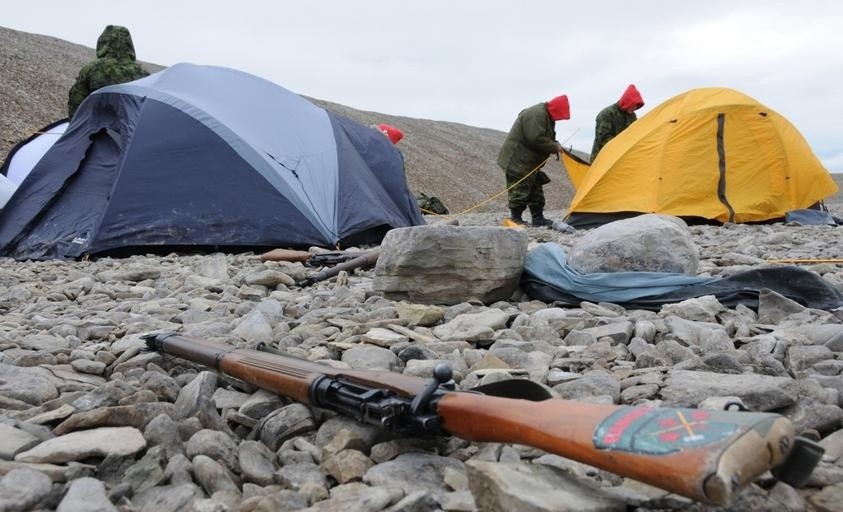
[
  {"x1": 494, "y1": 95, "x2": 570, "y2": 228},
  {"x1": 66, "y1": 24, "x2": 150, "y2": 126},
  {"x1": 588, "y1": 84, "x2": 644, "y2": 167}
]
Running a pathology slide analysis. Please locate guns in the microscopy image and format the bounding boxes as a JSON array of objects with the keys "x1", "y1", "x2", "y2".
[
  {"x1": 143, "y1": 322, "x2": 796, "y2": 509},
  {"x1": 260, "y1": 248, "x2": 372, "y2": 267},
  {"x1": 294, "y1": 219, "x2": 459, "y2": 288}
]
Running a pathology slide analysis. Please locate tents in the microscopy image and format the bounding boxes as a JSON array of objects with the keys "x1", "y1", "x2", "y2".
[
  {"x1": 548, "y1": 84, "x2": 828, "y2": 231},
  {"x1": 1, "y1": 58, "x2": 430, "y2": 257}
]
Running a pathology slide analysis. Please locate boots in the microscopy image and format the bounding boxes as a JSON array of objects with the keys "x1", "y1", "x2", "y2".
[
  {"x1": 510, "y1": 207, "x2": 528, "y2": 224},
  {"x1": 530, "y1": 208, "x2": 553, "y2": 226}
]
